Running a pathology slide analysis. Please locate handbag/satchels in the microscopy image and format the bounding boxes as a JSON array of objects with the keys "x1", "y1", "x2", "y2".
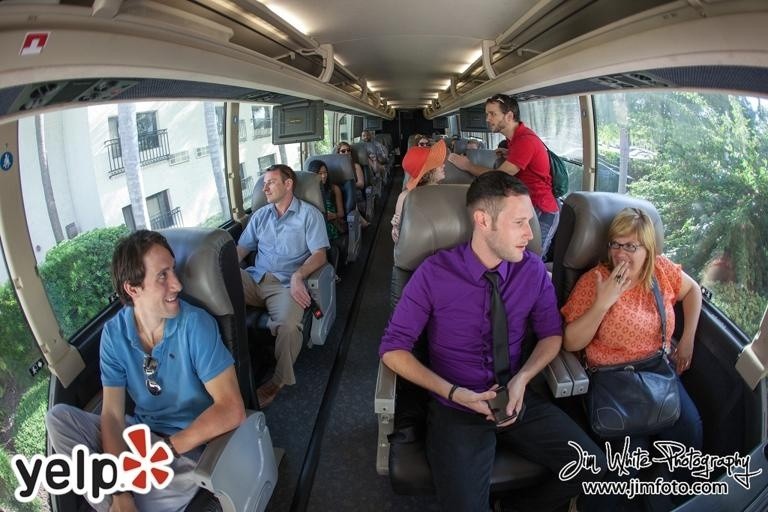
[{"x1": 587, "y1": 350, "x2": 681, "y2": 439}]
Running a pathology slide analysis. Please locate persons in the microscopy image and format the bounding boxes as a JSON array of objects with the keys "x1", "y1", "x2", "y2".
[
  {"x1": 390, "y1": 139, "x2": 447, "y2": 243},
  {"x1": 43, "y1": 229, "x2": 247, "y2": 512},
  {"x1": 308, "y1": 160, "x2": 345, "y2": 284},
  {"x1": 336, "y1": 142, "x2": 371, "y2": 230},
  {"x1": 378, "y1": 170, "x2": 608, "y2": 512},
  {"x1": 447, "y1": 94, "x2": 560, "y2": 259},
  {"x1": 359, "y1": 130, "x2": 388, "y2": 199},
  {"x1": 236, "y1": 164, "x2": 331, "y2": 411},
  {"x1": 414, "y1": 131, "x2": 481, "y2": 150},
  {"x1": 561, "y1": 208, "x2": 704, "y2": 512}
]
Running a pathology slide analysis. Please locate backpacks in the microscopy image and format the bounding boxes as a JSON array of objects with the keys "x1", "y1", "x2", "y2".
[{"x1": 546, "y1": 148, "x2": 569, "y2": 198}]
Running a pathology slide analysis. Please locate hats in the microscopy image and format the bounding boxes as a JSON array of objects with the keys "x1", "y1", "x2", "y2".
[{"x1": 399, "y1": 136, "x2": 447, "y2": 192}]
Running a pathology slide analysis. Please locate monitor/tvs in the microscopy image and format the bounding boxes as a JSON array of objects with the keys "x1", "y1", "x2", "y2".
[
  {"x1": 433, "y1": 118, "x2": 449, "y2": 128},
  {"x1": 272, "y1": 101, "x2": 324, "y2": 144},
  {"x1": 367, "y1": 118, "x2": 383, "y2": 130},
  {"x1": 460, "y1": 108, "x2": 491, "y2": 133}
]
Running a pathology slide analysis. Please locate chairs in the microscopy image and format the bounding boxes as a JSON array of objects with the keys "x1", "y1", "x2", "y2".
[{"x1": 83, "y1": 131, "x2": 707, "y2": 511}]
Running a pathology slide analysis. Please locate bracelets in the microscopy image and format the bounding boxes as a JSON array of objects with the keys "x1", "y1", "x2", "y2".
[
  {"x1": 448, "y1": 384, "x2": 460, "y2": 402},
  {"x1": 164, "y1": 436, "x2": 181, "y2": 459},
  {"x1": 110, "y1": 491, "x2": 134, "y2": 498}
]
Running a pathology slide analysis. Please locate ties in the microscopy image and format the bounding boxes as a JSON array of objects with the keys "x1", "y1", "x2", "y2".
[{"x1": 484, "y1": 272, "x2": 512, "y2": 386}]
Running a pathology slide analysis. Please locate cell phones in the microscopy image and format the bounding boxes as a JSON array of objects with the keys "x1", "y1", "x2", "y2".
[{"x1": 485, "y1": 385, "x2": 517, "y2": 425}]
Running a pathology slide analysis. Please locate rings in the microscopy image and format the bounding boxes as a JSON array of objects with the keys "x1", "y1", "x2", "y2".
[{"x1": 614, "y1": 274, "x2": 622, "y2": 282}]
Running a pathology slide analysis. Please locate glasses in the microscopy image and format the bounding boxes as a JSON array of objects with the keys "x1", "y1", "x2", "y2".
[
  {"x1": 339, "y1": 149, "x2": 352, "y2": 154},
  {"x1": 140, "y1": 349, "x2": 163, "y2": 398},
  {"x1": 417, "y1": 139, "x2": 430, "y2": 148},
  {"x1": 607, "y1": 239, "x2": 644, "y2": 252},
  {"x1": 490, "y1": 93, "x2": 506, "y2": 103}
]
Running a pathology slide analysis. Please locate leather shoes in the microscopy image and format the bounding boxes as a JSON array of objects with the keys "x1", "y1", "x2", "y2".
[{"x1": 255, "y1": 380, "x2": 283, "y2": 410}]
